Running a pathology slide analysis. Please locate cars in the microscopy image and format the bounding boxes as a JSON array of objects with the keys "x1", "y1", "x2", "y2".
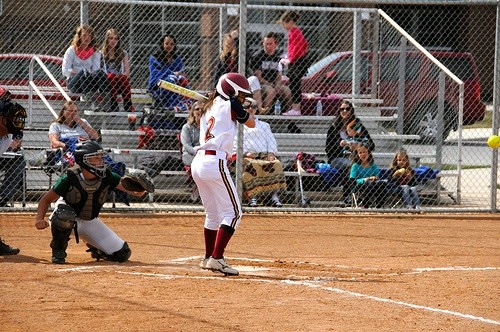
[{"x1": 1, "y1": 53, "x2": 122, "y2": 102}]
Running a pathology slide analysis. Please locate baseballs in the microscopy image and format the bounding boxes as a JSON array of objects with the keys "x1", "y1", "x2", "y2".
[{"x1": 487, "y1": 135, "x2": 500, "y2": 149}]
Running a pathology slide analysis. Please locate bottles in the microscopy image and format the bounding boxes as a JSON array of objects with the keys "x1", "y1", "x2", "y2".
[
  {"x1": 317, "y1": 100, "x2": 323, "y2": 115},
  {"x1": 127, "y1": 107, "x2": 137, "y2": 130},
  {"x1": 274, "y1": 100, "x2": 281, "y2": 115}
]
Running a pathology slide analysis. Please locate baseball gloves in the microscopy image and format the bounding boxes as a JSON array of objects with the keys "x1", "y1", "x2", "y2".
[{"x1": 121, "y1": 169, "x2": 155, "y2": 193}]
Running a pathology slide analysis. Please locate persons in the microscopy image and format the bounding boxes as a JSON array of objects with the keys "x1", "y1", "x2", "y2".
[
  {"x1": 382, "y1": 147, "x2": 421, "y2": 210},
  {"x1": 144, "y1": 35, "x2": 193, "y2": 111},
  {"x1": 99, "y1": 30, "x2": 133, "y2": 113},
  {"x1": 180, "y1": 101, "x2": 205, "y2": 201},
  {"x1": 349, "y1": 143, "x2": 391, "y2": 207},
  {"x1": 190, "y1": 74, "x2": 256, "y2": 278},
  {"x1": 279, "y1": 10, "x2": 310, "y2": 116},
  {"x1": 35, "y1": 141, "x2": 156, "y2": 264},
  {"x1": 326, "y1": 101, "x2": 376, "y2": 206},
  {"x1": 46, "y1": 101, "x2": 100, "y2": 166},
  {"x1": 232, "y1": 97, "x2": 286, "y2": 208},
  {"x1": 62, "y1": 26, "x2": 113, "y2": 113},
  {"x1": 0, "y1": 87, "x2": 27, "y2": 256},
  {"x1": 251, "y1": 32, "x2": 302, "y2": 134},
  {"x1": 343, "y1": 120, "x2": 370, "y2": 158},
  {"x1": 211, "y1": 32, "x2": 266, "y2": 114}
]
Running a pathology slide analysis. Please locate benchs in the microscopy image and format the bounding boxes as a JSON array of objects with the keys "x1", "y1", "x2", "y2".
[{"x1": 9, "y1": 89, "x2": 459, "y2": 206}]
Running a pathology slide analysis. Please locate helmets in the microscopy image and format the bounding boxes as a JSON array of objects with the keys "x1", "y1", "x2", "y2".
[
  {"x1": 74, "y1": 141, "x2": 107, "y2": 178},
  {"x1": 216, "y1": 72, "x2": 254, "y2": 101},
  {"x1": 0, "y1": 87, "x2": 12, "y2": 101}
]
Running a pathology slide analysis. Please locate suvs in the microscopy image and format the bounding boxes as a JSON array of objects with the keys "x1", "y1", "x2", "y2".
[{"x1": 277, "y1": 46, "x2": 488, "y2": 145}]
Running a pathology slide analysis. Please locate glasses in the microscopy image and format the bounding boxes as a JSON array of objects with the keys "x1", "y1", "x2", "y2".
[
  {"x1": 246, "y1": 104, "x2": 259, "y2": 109},
  {"x1": 339, "y1": 107, "x2": 351, "y2": 111}
]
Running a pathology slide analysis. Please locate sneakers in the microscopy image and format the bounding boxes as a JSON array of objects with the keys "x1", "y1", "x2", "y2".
[
  {"x1": 406, "y1": 205, "x2": 413, "y2": 209},
  {"x1": 271, "y1": 198, "x2": 283, "y2": 207},
  {"x1": 283, "y1": 109, "x2": 301, "y2": 116},
  {"x1": 415, "y1": 205, "x2": 420, "y2": 209},
  {"x1": 0, "y1": 238, "x2": 20, "y2": 256},
  {"x1": 248, "y1": 198, "x2": 258, "y2": 206},
  {"x1": 205, "y1": 256, "x2": 239, "y2": 275},
  {"x1": 200, "y1": 256, "x2": 220, "y2": 271},
  {"x1": 52, "y1": 249, "x2": 67, "y2": 263}
]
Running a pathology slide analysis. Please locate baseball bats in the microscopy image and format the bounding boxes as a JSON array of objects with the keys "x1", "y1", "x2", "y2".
[{"x1": 157, "y1": 79, "x2": 209, "y2": 104}]
